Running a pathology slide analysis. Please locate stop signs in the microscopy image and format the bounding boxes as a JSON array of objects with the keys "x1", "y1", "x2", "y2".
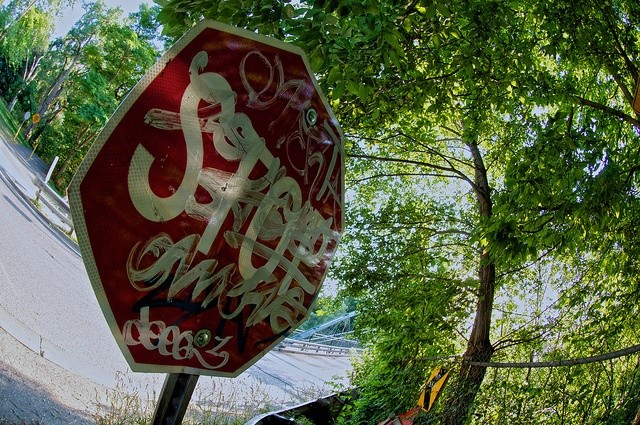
[{"x1": 68, "y1": 21, "x2": 344, "y2": 376}]
